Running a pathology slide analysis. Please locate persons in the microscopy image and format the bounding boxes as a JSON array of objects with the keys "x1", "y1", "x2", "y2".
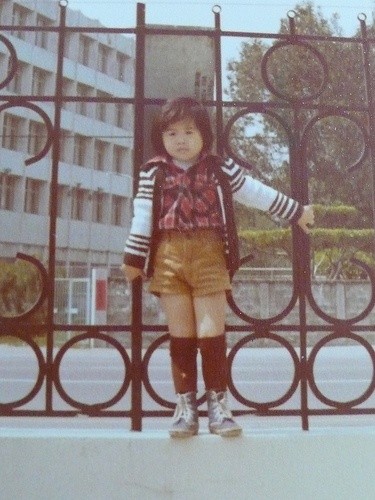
[{"x1": 117, "y1": 95, "x2": 317, "y2": 438}]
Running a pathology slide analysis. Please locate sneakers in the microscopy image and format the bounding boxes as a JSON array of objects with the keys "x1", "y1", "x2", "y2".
[
  {"x1": 168, "y1": 392, "x2": 199, "y2": 437},
  {"x1": 206, "y1": 391, "x2": 242, "y2": 436}
]
